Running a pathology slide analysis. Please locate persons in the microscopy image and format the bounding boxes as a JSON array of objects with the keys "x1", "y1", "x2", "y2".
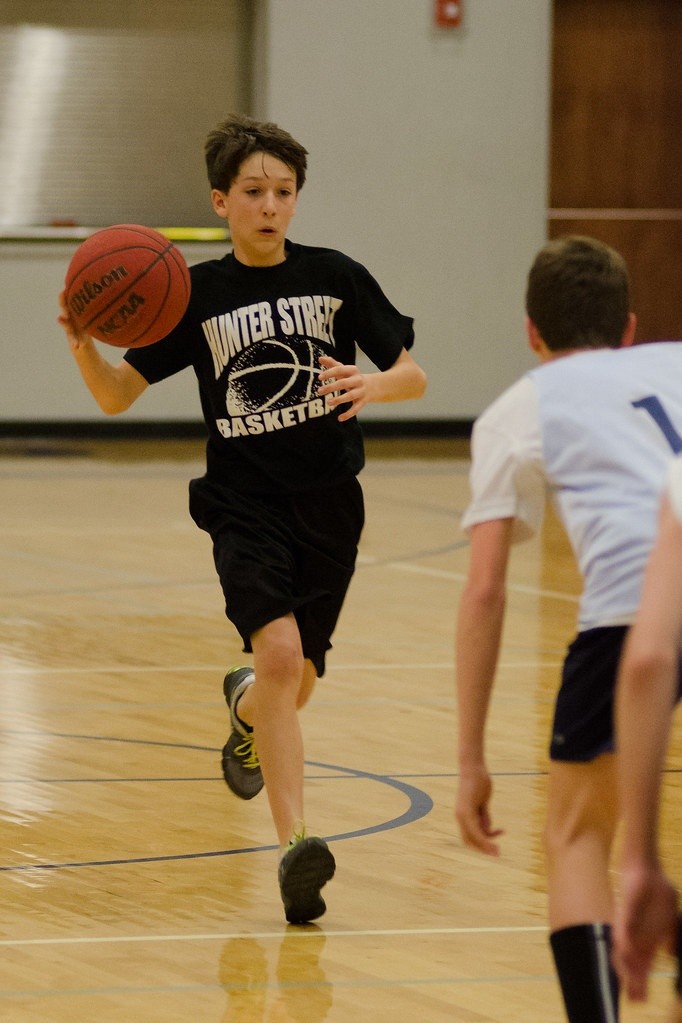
[
  {"x1": 456, "y1": 235, "x2": 682, "y2": 1023},
  {"x1": 218, "y1": 923, "x2": 333, "y2": 1023},
  {"x1": 612, "y1": 452, "x2": 682, "y2": 1023},
  {"x1": 57, "y1": 111, "x2": 426, "y2": 926}
]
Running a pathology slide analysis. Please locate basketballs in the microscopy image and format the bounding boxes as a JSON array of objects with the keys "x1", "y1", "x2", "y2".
[{"x1": 64, "y1": 223, "x2": 191, "y2": 348}]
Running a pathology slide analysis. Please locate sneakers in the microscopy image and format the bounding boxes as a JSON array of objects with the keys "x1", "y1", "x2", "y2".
[
  {"x1": 222, "y1": 665, "x2": 265, "y2": 800},
  {"x1": 278, "y1": 819, "x2": 336, "y2": 923}
]
